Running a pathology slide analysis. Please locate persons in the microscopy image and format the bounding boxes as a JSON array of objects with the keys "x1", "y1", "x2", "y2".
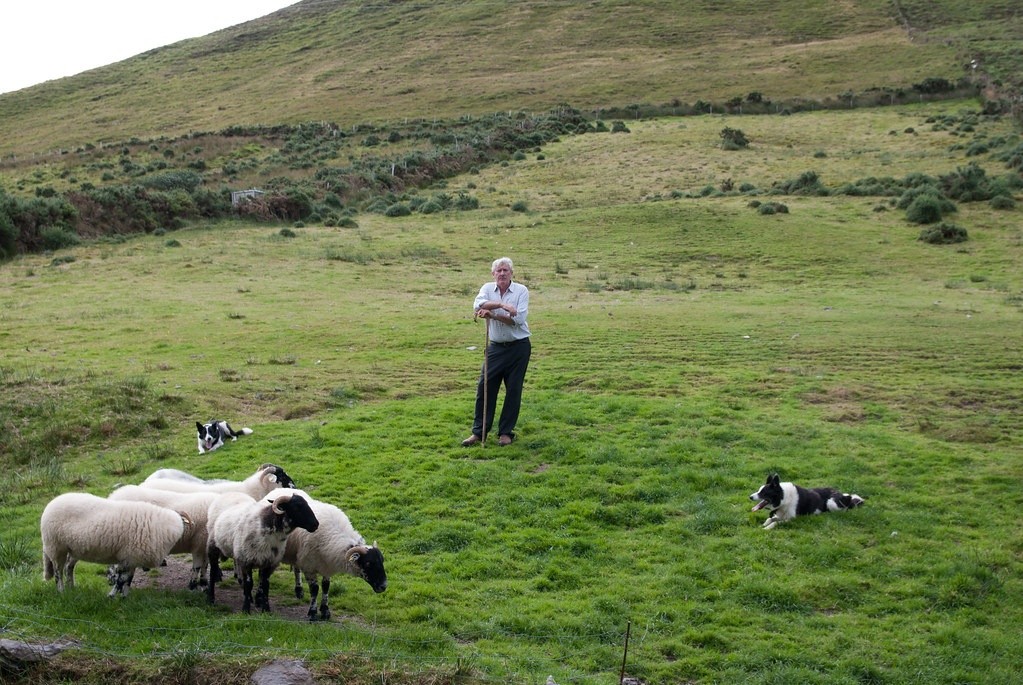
[{"x1": 462, "y1": 257, "x2": 532, "y2": 445}]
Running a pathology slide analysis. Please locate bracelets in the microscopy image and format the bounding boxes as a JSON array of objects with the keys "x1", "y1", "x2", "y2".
[{"x1": 493, "y1": 312, "x2": 497, "y2": 320}]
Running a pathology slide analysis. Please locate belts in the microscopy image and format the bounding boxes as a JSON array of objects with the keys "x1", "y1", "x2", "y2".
[{"x1": 490, "y1": 336, "x2": 529, "y2": 347}]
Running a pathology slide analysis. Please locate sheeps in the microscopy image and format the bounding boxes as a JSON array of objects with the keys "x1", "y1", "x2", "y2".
[{"x1": 40, "y1": 463, "x2": 388, "y2": 621}]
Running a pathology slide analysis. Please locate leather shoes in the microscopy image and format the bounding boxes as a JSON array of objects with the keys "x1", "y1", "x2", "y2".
[
  {"x1": 461, "y1": 433, "x2": 480, "y2": 445},
  {"x1": 498, "y1": 434, "x2": 512, "y2": 445}
]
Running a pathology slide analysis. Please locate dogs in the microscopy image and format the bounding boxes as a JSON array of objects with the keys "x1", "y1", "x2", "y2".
[
  {"x1": 196, "y1": 419, "x2": 253, "y2": 455},
  {"x1": 749, "y1": 473, "x2": 869, "y2": 530}
]
